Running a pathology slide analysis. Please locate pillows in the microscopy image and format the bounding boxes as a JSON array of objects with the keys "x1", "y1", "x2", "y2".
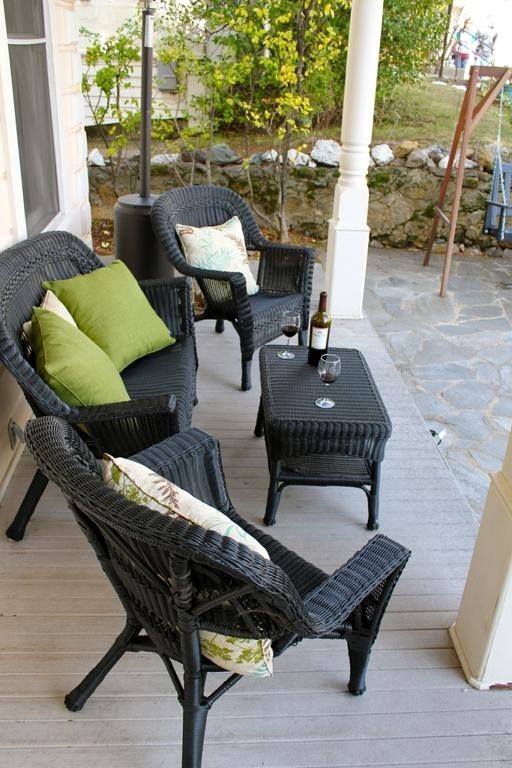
[
  {"x1": 167, "y1": 214, "x2": 262, "y2": 318},
  {"x1": 19, "y1": 255, "x2": 178, "y2": 434},
  {"x1": 95, "y1": 446, "x2": 276, "y2": 684}
]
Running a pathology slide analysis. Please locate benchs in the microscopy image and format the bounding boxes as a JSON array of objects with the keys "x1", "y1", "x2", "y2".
[{"x1": 0, "y1": 230, "x2": 200, "y2": 543}]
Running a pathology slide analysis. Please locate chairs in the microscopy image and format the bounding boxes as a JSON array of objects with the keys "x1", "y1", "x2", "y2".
[
  {"x1": 23, "y1": 415, "x2": 414, "y2": 767},
  {"x1": 153, "y1": 182, "x2": 317, "y2": 394}
]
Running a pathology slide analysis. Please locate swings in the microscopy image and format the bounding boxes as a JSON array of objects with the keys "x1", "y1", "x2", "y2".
[{"x1": 483, "y1": 86, "x2": 512, "y2": 242}]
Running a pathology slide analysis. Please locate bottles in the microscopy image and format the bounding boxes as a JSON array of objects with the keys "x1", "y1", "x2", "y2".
[{"x1": 308, "y1": 291, "x2": 332, "y2": 367}]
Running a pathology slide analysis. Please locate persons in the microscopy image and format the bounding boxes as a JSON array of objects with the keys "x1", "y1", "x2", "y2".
[{"x1": 449, "y1": 16, "x2": 477, "y2": 79}]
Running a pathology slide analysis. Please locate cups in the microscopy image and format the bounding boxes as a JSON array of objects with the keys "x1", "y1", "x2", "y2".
[
  {"x1": 277, "y1": 310, "x2": 300, "y2": 360},
  {"x1": 314, "y1": 354, "x2": 341, "y2": 409}
]
